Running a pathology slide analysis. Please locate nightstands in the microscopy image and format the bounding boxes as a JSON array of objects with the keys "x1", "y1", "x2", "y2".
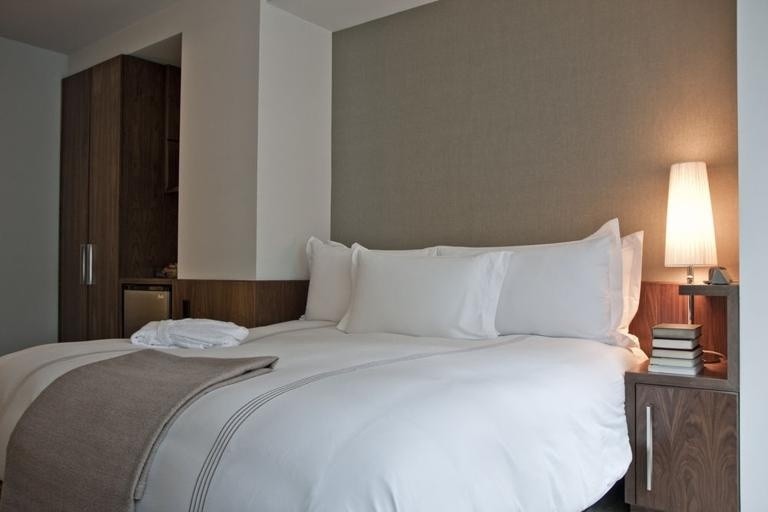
[{"x1": 620, "y1": 280, "x2": 740, "y2": 512}]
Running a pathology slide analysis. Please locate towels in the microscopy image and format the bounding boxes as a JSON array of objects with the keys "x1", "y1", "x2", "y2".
[{"x1": 129, "y1": 317, "x2": 249, "y2": 353}]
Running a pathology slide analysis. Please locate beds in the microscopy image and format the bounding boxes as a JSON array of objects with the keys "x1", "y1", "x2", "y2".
[{"x1": 0, "y1": 316, "x2": 650, "y2": 512}]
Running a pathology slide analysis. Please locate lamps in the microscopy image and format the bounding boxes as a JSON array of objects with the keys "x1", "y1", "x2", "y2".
[{"x1": 658, "y1": 158, "x2": 727, "y2": 364}]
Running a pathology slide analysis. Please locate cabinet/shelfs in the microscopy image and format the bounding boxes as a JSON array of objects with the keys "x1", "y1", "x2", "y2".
[{"x1": 57, "y1": 54, "x2": 181, "y2": 342}]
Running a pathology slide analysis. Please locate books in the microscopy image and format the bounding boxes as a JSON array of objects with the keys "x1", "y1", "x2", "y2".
[
  {"x1": 652, "y1": 337, "x2": 700, "y2": 350},
  {"x1": 653, "y1": 322, "x2": 703, "y2": 339},
  {"x1": 648, "y1": 354, "x2": 703, "y2": 367},
  {"x1": 647, "y1": 363, "x2": 704, "y2": 375},
  {"x1": 651, "y1": 347, "x2": 703, "y2": 359}
]
